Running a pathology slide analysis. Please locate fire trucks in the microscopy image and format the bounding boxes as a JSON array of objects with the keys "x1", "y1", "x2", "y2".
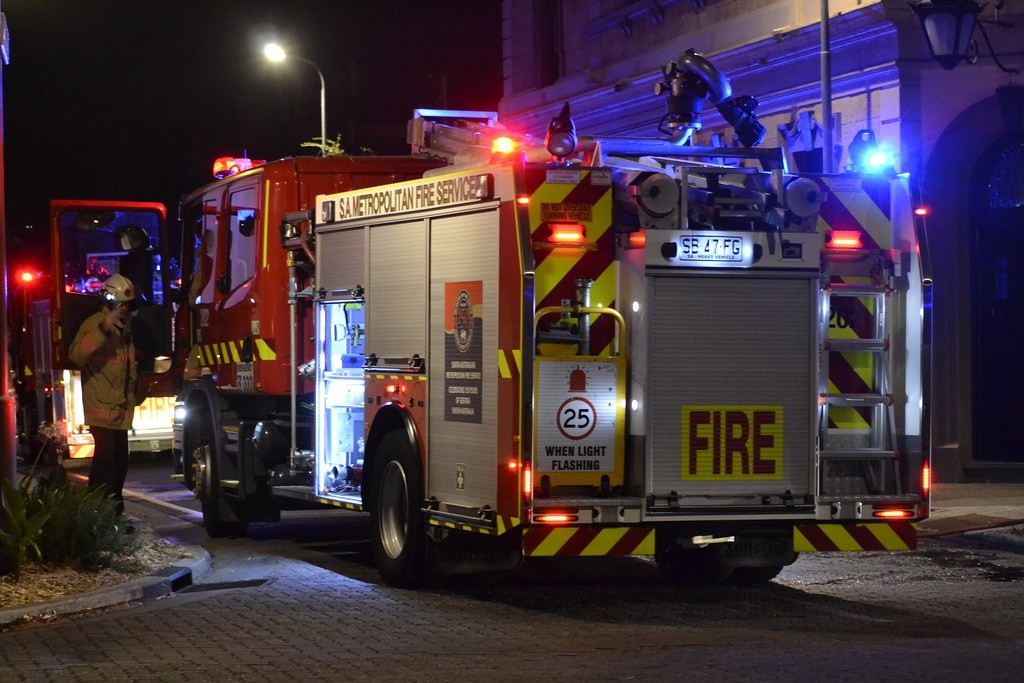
[{"x1": 14, "y1": 48, "x2": 932, "y2": 585}]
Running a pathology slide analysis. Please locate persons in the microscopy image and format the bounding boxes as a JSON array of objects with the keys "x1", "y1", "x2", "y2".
[{"x1": 67, "y1": 273, "x2": 137, "y2": 537}]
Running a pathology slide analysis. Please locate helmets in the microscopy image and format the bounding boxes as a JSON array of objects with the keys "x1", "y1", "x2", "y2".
[{"x1": 102, "y1": 274, "x2": 135, "y2": 302}]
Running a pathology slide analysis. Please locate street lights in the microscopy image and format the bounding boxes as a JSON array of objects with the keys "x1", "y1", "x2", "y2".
[{"x1": 263, "y1": 43, "x2": 325, "y2": 156}]
